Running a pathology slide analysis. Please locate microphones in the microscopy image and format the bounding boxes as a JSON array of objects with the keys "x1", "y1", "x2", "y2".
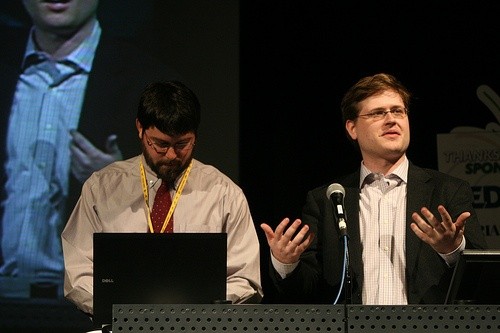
[{"x1": 326, "y1": 183, "x2": 348, "y2": 238}]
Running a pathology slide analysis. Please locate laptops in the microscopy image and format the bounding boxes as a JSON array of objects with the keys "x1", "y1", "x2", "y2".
[{"x1": 90, "y1": 232, "x2": 227, "y2": 330}]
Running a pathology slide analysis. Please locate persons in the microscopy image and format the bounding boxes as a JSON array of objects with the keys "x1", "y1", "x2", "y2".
[
  {"x1": 266, "y1": 74, "x2": 488, "y2": 305},
  {"x1": 0, "y1": 0, "x2": 152, "y2": 303},
  {"x1": 59, "y1": 82, "x2": 266, "y2": 321}
]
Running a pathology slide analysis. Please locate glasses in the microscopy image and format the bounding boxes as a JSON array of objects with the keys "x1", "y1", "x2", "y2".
[
  {"x1": 357, "y1": 108, "x2": 410, "y2": 121},
  {"x1": 144, "y1": 132, "x2": 195, "y2": 154}
]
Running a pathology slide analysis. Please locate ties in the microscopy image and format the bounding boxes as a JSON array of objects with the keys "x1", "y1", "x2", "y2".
[{"x1": 147, "y1": 175, "x2": 176, "y2": 233}]
